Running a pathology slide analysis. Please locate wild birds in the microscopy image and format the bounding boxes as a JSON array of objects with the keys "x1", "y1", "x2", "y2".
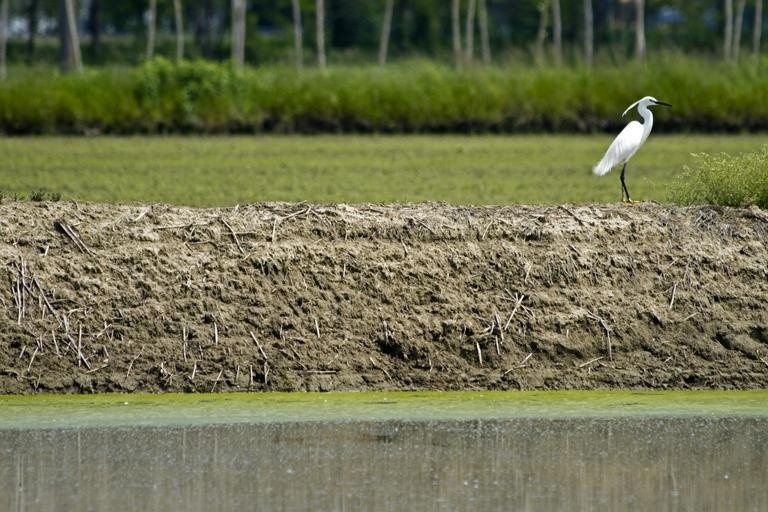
[{"x1": 591, "y1": 95, "x2": 673, "y2": 204}]
